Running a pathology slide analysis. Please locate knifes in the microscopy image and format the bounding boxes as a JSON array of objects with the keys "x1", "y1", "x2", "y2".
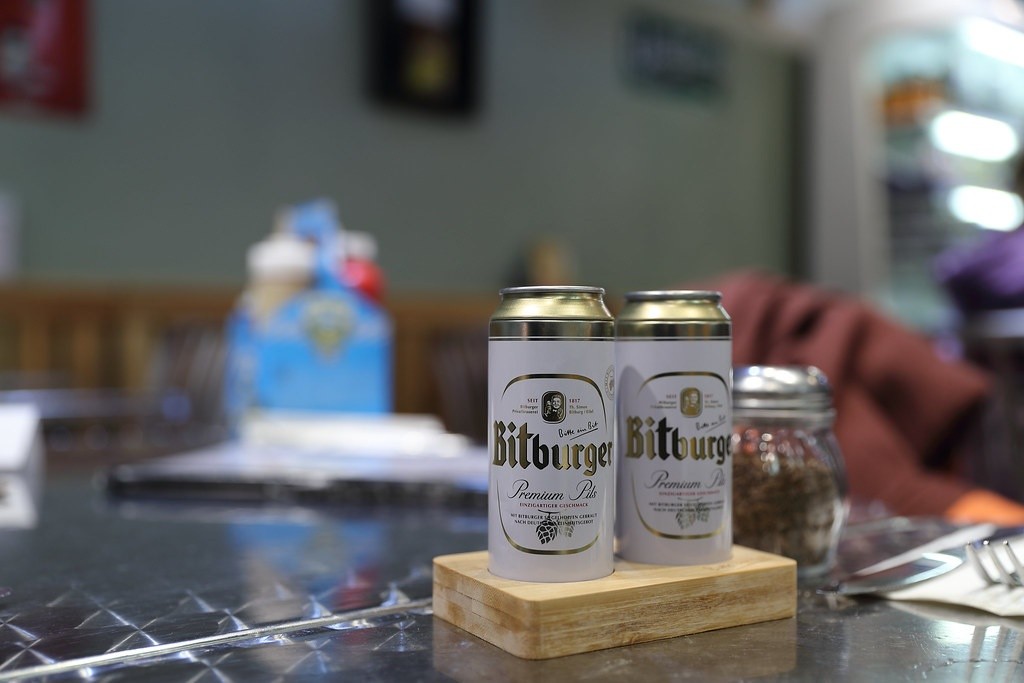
[{"x1": 815, "y1": 522, "x2": 997, "y2": 596}]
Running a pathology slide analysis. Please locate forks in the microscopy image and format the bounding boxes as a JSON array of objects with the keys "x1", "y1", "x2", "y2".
[{"x1": 964, "y1": 540, "x2": 1023, "y2": 587}]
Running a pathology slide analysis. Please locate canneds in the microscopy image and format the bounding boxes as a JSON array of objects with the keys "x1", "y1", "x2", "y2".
[{"x1": 486, "y1": 283, "x2": 737, "y2": 583}]
